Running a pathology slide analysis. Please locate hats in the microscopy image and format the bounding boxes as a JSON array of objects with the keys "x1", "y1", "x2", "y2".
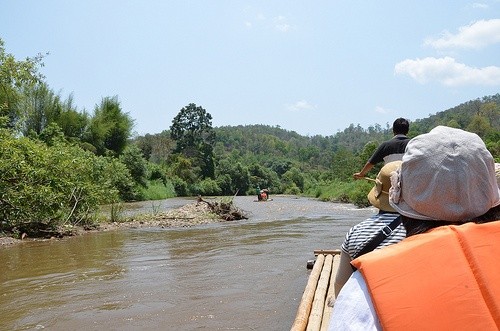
[
  {"x1": 367, "y1": 160, "x2": 403, "y2": 214},
  {"x1": 388, "y1": 124, "x2": 500, "y2": 221}
]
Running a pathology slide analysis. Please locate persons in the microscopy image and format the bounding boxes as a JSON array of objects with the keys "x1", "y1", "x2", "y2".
[
  {"x1": 352, "y1": 118, "x2": 412, "y2": 181},
  {"x1": 334, "y1": 160, "x2": 407, "y2": 301},
  {"x1": 326, "y1": 125, "x2": 500, "y2": 331}
]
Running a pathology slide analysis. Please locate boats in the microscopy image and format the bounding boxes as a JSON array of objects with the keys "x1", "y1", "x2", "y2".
[{"x1": 252, "y1": 198, "x2": 274, "y2": 202}]
